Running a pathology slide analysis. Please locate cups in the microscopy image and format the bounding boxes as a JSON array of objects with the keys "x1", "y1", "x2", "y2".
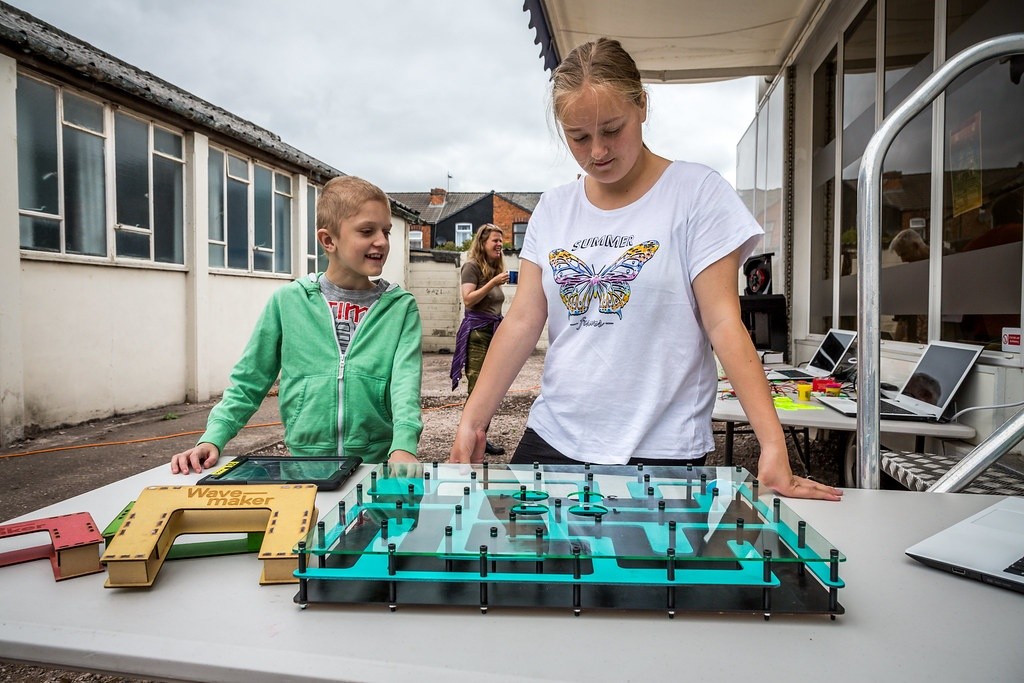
[
  {"x1": 826, "y1": 383, "x2": 841, "y2": 397},
  {"x1": 797, "y1": 383, "x2": 812, "y2": 401},
  {"x1": 507, "y1": 271, "x2": 518, "y2": 284}
]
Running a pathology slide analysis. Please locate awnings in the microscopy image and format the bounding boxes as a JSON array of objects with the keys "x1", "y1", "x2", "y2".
[{"x1": 523, "y1": 0, "x2": 821, "y2": 85}]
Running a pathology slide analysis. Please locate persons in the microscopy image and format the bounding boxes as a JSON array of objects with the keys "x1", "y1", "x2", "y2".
[
  {"x1": 447, "y1": 38, "x2": 844, "y2": 503},
  {"x1": 889, "y1": 225, "x2": 991, "y2": 343},
  {"x1": 461, "y1": 222, "x2": 510, "y2": 457},
  {"x1": 171, "y1": 174, "x2": 424, "y2": 479}
]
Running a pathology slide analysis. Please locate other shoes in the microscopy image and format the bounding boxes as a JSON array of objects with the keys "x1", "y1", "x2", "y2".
[{"x1": 485, "y1": 441, "x2": 505, "y2": 455}]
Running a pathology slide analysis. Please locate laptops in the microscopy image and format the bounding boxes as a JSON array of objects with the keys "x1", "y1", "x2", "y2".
[
  {"x1": 766, "y1": 329, "x2": 858, "y2": 381},
  {"x1": 816, "y1": 340, "x2": 985, "y2": 423},
  {"x1": 905, "y1": 496, "x2": 1024, "y2": 595}
]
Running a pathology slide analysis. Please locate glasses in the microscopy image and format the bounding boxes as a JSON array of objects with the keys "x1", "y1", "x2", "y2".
[{"x1": 483, "y1": 223, "x2": 499, "y2": 232}]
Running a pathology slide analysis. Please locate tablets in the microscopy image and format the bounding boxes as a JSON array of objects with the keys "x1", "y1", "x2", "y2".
[{"x1": 196, "y1": 455, "x2": 363, "y2": 491}]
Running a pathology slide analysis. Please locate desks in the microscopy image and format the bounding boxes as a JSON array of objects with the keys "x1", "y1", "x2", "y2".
[
  {"x1": 710, "y1": 354, "x2": 976, "y2": 489},
  {"x1": 0, "y1": 452, "x2": 1024, "y2": 683}
]
